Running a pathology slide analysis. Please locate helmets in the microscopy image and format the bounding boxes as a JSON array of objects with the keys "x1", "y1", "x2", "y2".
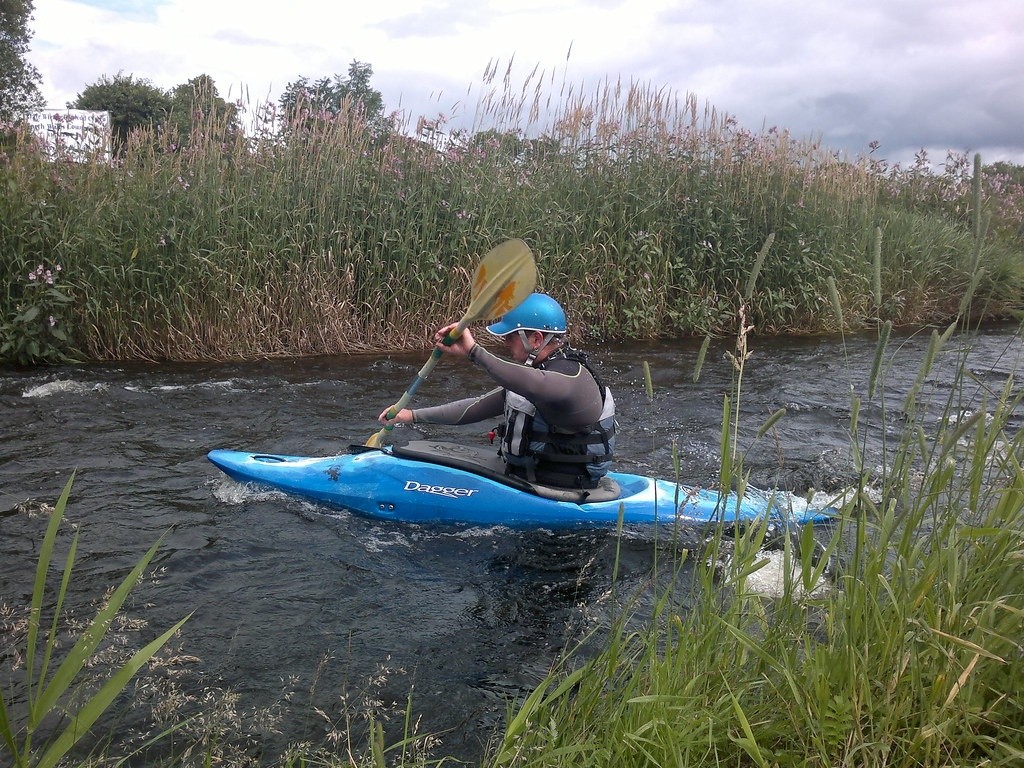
[{"x1": 486, "y1": 293, "x2": 567, "y2": 336}]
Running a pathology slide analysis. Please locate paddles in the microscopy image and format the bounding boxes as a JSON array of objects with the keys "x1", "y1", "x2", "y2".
[{"x1": 366, "y1": 238, "x2": 540, "y2": 448}]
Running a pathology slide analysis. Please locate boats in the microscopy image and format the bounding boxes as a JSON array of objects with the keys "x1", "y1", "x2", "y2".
[{"x1": 209, "y1": 441, "x2": 840, "y2": 532}]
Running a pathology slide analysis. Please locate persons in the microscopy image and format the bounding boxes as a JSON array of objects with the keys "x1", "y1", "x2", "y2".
[{"x1": 378, "y1": 294, "x2": 615, "y2": 488}]
